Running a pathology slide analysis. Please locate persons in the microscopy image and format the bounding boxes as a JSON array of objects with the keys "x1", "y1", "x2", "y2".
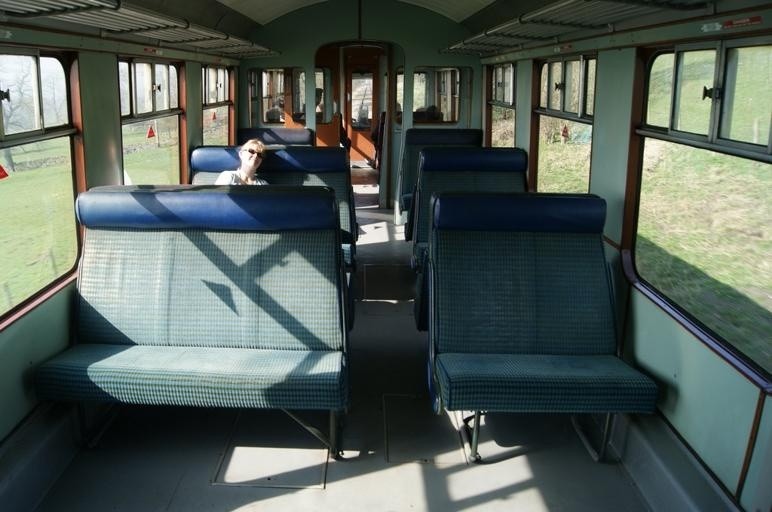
[
  {"x1": 214, "y1": 137, "x2": 268, "y2": 186},
  {"x1": 265, "y1": 108, "x2": 280, "y2": 123}
]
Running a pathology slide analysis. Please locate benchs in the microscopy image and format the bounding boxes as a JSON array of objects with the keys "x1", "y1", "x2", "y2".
[
  {"x1": 399, "y1": 146, "x2": 529, "y2": 333},
  {"x1": 28, "y1": 182, "x2": 352, "y2": 462},
  {"x1": 426, "y1": 189, "x2": 662, "y2": 469},
  {"x1": 238, "y1": 127, "x2": 314, "y2": 147},
  {"x1": 189, "y1": 144, "x2": 358, "y2": 333},
  {"x1": 372, "y1": 111, "x2": 445, "y2": 185},
  {"x1": 393, "y1": 127, "x2": 485, "y2": 217}
]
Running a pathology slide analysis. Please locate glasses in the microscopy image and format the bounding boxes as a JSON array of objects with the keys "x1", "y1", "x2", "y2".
[{"x1": 245, "y1": 148, "x2": 264, "y2": 161}]
